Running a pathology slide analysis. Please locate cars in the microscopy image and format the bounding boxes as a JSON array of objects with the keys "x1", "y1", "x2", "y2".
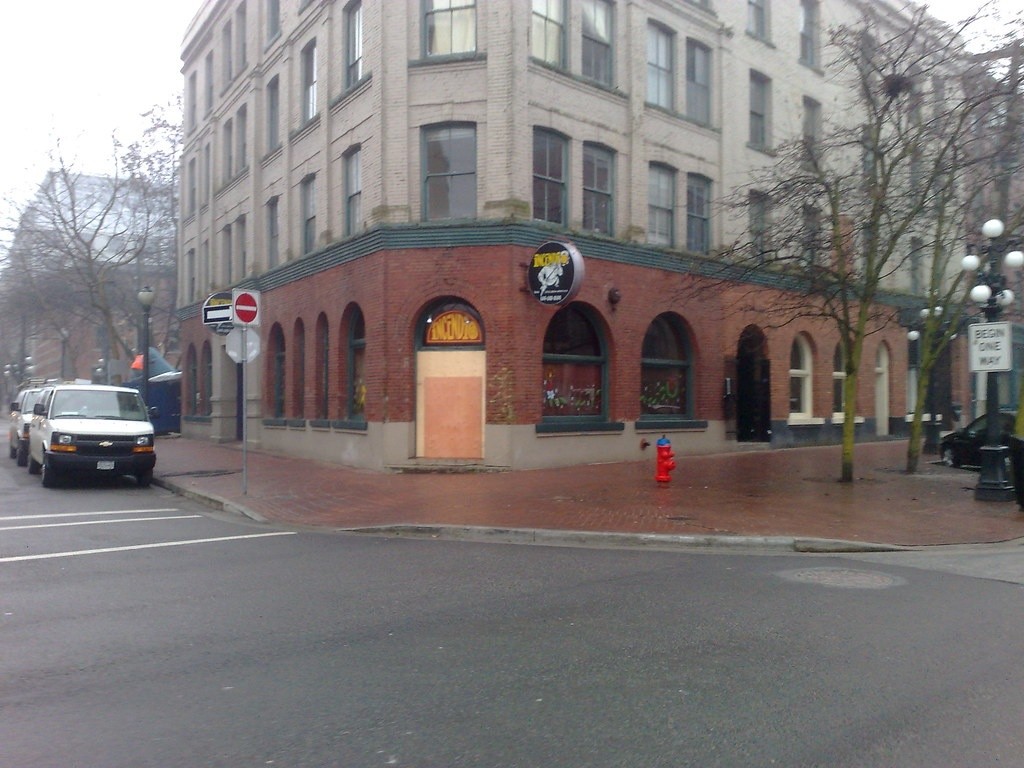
[
  {"x1": 941, "y1": 408, "x2": 1017, "y2": 469},
  {"x1": 150, "y1": 372, "x2": 182, "y2": 435}
]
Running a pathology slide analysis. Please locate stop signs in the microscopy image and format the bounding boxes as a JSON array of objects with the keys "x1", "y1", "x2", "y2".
[{"x1": 232, "y1": 288, "x2": 262, "y2": 328}]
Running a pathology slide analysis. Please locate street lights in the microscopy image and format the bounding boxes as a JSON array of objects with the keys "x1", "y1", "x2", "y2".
[
  {"x1": 57, "y1": 328, "x2": 70, "y2": 378},
  {"x1": 963, "y1": 219, "x2": 1024, "y2": 503},
  {"x1": 138, "y1": 286, "x2": 155, "y2": 409},
  {"x1": 907, "y1": 307, "x2": 958, "y2": 454}
]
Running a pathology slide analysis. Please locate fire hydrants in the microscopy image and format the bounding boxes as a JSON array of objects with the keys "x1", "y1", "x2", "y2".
[{"x1": 655, "y1": 434, "x2": 676, "y2": 486}]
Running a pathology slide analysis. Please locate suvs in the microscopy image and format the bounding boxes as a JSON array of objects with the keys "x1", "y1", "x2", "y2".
[{"x1": 10, "y1": 378, "x2": 159, "y2": 487}]
